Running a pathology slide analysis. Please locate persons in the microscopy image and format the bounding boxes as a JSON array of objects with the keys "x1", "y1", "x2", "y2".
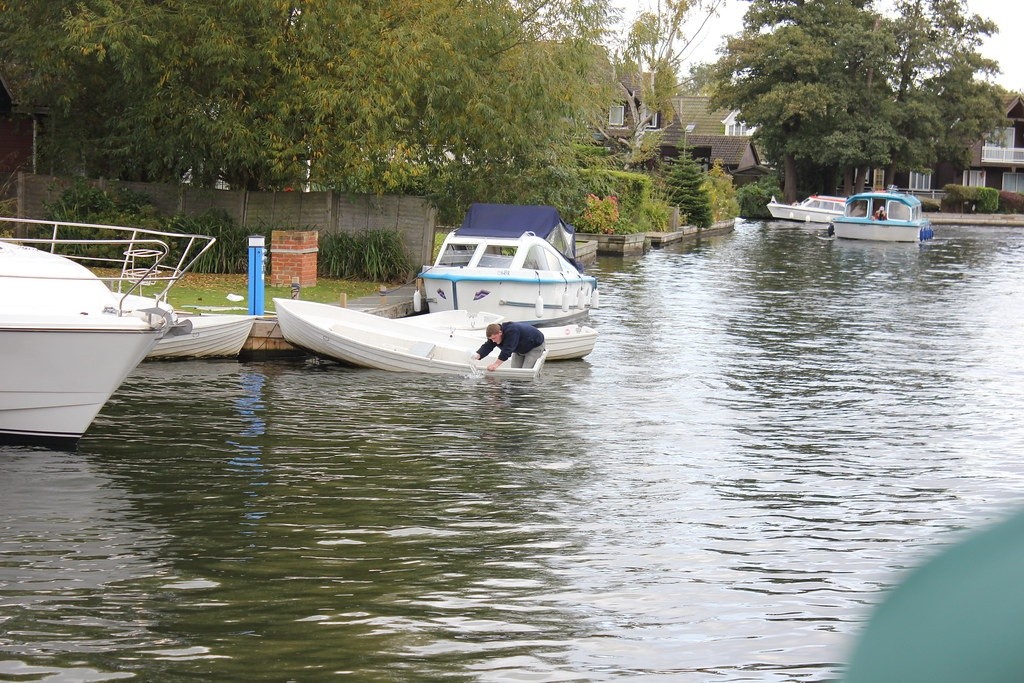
[
  {"x1": 875, "y1": 206, "x2": 887, "y2": 220},
  {"x1": 471, "y1": 321, "x2": 545, "y2": 372}
]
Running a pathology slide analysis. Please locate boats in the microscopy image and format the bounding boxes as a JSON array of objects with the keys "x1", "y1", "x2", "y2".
[
  {"x1": 766, "y1": 191, "x2": 847, "y2": 225},
  {"x1": 0, "y1": 216, "x2": 217, "y2": 444},
  {"x1": 271, "y1": 297, "x2": 548, "y2": 383},
  {"x1": 831, "y1": 185, "x2": 932, "y2": 243},
  {"x1": 387, "y1": 307, "x2": 598, "y2": 363},
  {"x1": 145, "y1": 312, "x2": 258, "y2": 359},
  {"x1": 417, "y1": 201, "x2": 600, "y2": 329}
]
